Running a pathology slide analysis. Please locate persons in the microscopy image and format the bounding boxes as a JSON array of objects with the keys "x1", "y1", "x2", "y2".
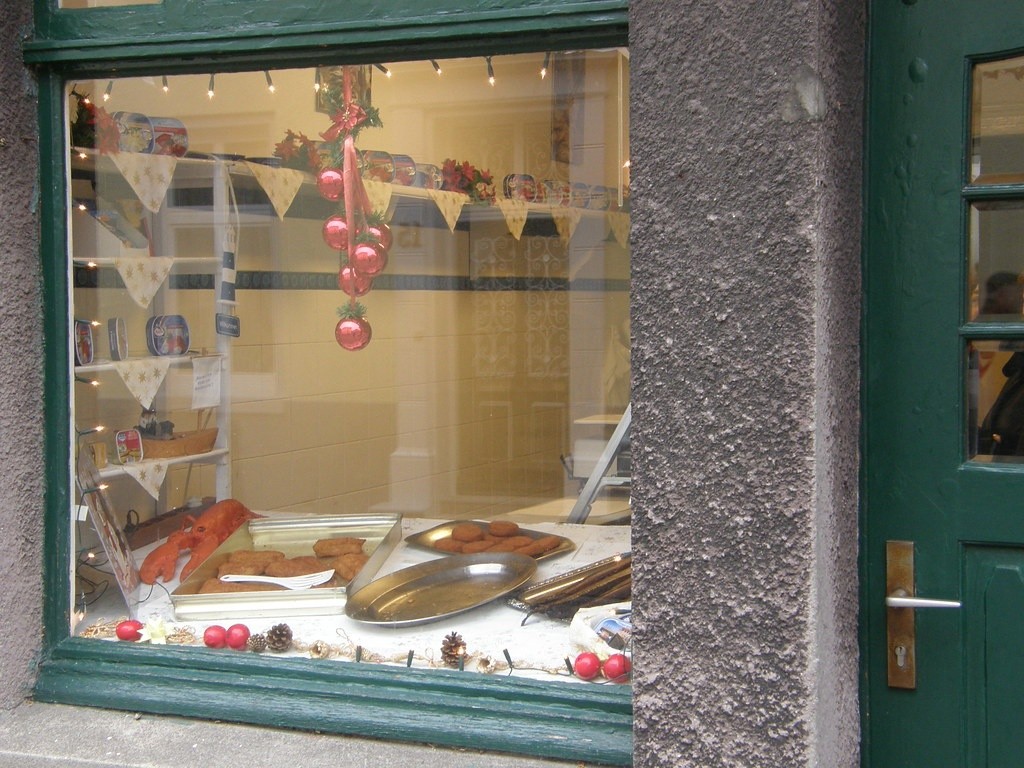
[{"x1": 978, "y1": 273, "x2": 1024, "y2": 457}]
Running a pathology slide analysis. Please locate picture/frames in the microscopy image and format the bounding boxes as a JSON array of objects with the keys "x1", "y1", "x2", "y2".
[{"x1": 314, "y1": 64, "x2": 372, "y2": 113}]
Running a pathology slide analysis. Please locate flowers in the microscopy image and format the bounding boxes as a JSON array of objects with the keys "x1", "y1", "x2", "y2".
[
  {"x1": 71, "y1": 97, "x2": 121, "y2": 156},
  {"x1": 275, "y1": 128, "x2": 321, "y2": 171},
  {"x1": 440, "y1": 157, "x2": 496, "y2": 209}
]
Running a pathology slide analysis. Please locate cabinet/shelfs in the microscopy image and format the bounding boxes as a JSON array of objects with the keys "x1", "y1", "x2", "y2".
[{"x1": 71, "y1": 147, "x2": 234, "y2": 503}]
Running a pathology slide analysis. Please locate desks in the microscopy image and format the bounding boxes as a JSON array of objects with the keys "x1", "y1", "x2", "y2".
[{"x1": 69, "y1": 496, "x2": 632, "y2": 687}]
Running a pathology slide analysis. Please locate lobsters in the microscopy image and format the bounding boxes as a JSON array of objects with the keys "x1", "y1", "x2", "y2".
[{"x1": 139, "y1": 498, "x2": 270, "y2": 584}]
